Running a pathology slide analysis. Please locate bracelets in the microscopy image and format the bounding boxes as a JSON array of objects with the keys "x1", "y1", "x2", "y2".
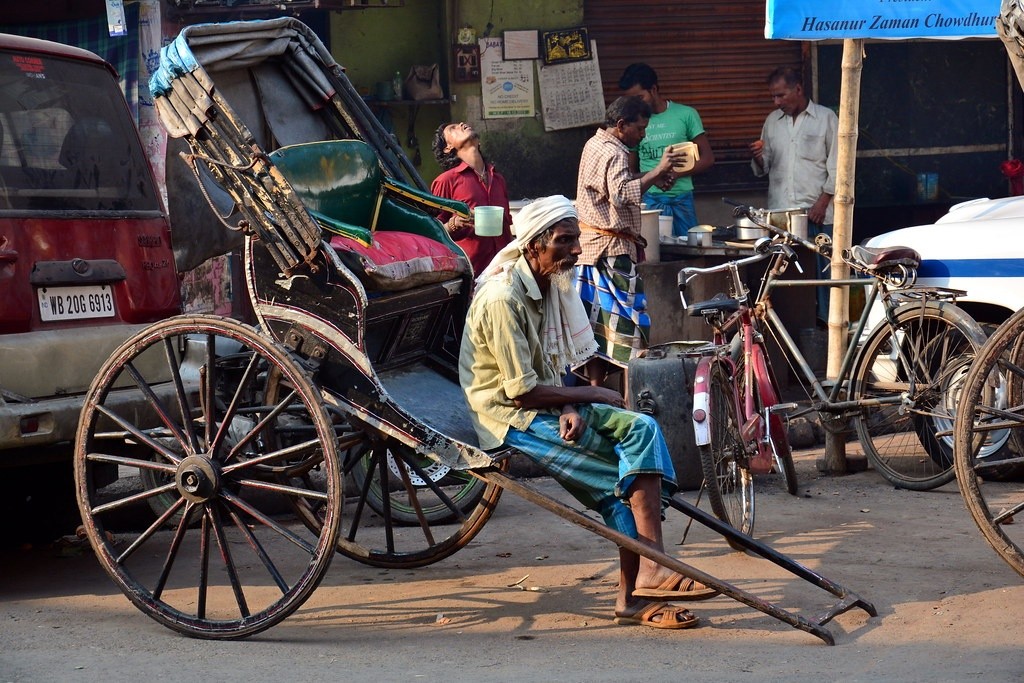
[{"x1": 449, "y1": 217, "x2": 458, "y2": 231}]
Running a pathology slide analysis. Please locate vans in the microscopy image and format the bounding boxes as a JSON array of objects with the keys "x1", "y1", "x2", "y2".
[{"x1": 0, "y1": 32, "x2": 210, "y2": 446}]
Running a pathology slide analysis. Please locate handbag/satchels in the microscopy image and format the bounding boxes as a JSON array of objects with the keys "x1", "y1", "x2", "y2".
[
  {"x1": 403, "y1": 62, "x2": 445, "y2": 101},
  {"x1": 632, "y1": 233, "x2": 648, "y2": 264}
]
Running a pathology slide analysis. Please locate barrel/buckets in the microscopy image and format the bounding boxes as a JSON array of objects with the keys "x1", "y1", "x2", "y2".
[
  {"x1": 641, "y1": 210, "x2": 673, "y2": 262},
  {"x1": 628, "y1": 341, "x2": 725, "y2": 490}
]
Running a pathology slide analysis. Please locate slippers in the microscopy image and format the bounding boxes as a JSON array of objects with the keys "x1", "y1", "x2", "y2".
[
  {"x1": 630, "y1": 571, "x2": 723, "y2": 601},
  {"x1": 613, "y1": 600, "x2": 700, "y2": 629}
]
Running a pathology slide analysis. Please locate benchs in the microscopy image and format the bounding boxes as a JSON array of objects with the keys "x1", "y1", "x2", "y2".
[{"x1": 269, "y1": 140, "x2": 471, "y2": 299}]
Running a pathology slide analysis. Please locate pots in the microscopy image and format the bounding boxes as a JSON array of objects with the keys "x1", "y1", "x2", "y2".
[{"x1": 736, "y1": 207, "x2": 811, "y2": 240}]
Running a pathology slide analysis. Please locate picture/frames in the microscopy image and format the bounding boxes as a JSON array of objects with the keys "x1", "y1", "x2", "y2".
[
  {"x1": 540, "y1": 25, "x2": 591, "y2": 64},
  {"x1": 452, "y1": 44, "x2": 481, "y2": 83},
  {"x1": 501, "y1": 28, "x2": 540, "y2": 61}
]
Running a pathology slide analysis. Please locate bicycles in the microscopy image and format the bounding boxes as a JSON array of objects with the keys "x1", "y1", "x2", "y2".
[
  {"x1": 717, "y1": 197, "x2": 999, "y2": 494},
  {"x1": 676, "y1": 233, "x2": 804, "y2": 552}
]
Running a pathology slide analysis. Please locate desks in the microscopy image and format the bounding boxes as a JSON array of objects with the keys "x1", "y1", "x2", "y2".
[{"x1": 634, "y1": 235, "x2": 818, "y2": 398}]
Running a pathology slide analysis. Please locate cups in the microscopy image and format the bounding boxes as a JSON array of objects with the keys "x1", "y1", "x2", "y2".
[
  {"x1": 688, "y1": 232, "x2": 697, "y2": 247},
  {"x1": 790, "y1": 214, "x2": 808, "y2": 241},
  {"x1": 702, "y1": 232, "x2": 713, "y2": 247},
  {"x1": 464, "y1": 206, "x2": 504, "y2": 236},
  {"x1": 665, "y1": 142, "x2": 700, "y2": 172}
]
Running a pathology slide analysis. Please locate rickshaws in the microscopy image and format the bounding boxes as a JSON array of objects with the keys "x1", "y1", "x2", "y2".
[
  {"x1": 74, "y1": 17, "x2": 877, "y2": 646},
  {"x1": 952, "y1": 308, "x2": 1024, "y2": 578}
]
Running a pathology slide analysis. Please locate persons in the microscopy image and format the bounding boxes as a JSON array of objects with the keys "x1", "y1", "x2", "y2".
[
  {"x1": 750, "y1": 67, "x2": 839, "y2": 325},
  {"x1": 431, "y1": 122, "x2": 514, "y2": 278},
  {"x1": 459, "y1": 194, "x2": 720, "y2": 628},
  {"x1": 573, "y1": 95, "x2": 689, "y2": 387},
  {"x1": 618, "y1": 63, "x2": 715, "y2": 237}
]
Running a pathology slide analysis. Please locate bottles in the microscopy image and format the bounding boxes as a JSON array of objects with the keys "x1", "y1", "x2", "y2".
[{"x1": 393, "y1": 70, "x2": 403, "y2": 101}]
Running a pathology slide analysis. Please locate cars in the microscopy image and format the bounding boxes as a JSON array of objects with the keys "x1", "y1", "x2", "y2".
[{"x1": 851, "y1": 195, "x2": 1024, "y2": 481}]
